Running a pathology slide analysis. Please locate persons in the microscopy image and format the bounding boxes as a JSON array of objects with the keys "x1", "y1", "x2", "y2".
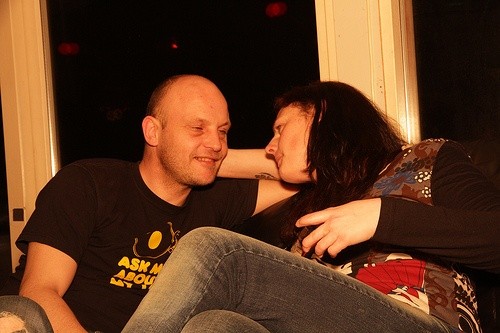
[
  {"x1": 0, "y1": 75, "x2": 301, "y2": 333},
  {"x1": 121, "y1": 80, "x2": 499, "y2": 333}
]
